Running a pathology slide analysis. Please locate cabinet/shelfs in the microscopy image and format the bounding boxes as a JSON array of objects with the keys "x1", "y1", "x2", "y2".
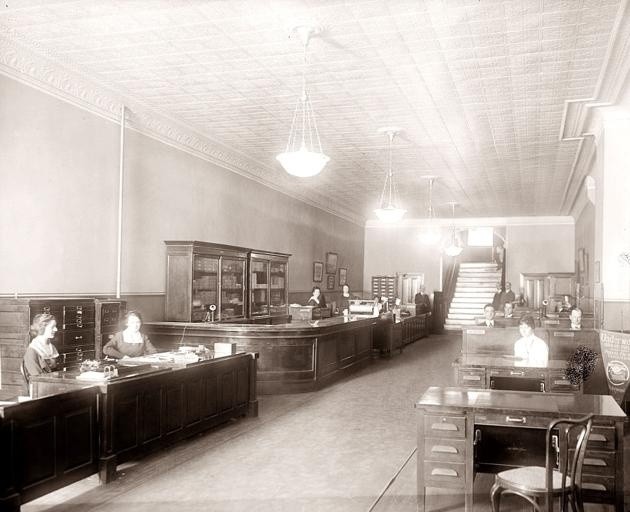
[
  {"x1": 372, "y1": 276, "x2": 397, "y2": 304},
  {"x1": 0, "y1": 386, "x2": 101, "y2": 511},
  {"x1": 1, "y1": 297, "x2": 96, "y2": 401},
  {"x1": 251, "y1": 248, "x2": 293, "y2": 325},
  {"x1": 163, "y1": 240, "x2": 250, "y2": 321},
  {"x1": 95, "y1": 299, "x2": 128, "y2": 360},
  {"x1": 30, "y1": 352, "x2": 259, "y2": 481}
]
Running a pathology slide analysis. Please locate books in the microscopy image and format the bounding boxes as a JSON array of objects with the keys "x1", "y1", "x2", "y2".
[{"x1": 193, "y1": 257, "x2": 284, "y2": 322}]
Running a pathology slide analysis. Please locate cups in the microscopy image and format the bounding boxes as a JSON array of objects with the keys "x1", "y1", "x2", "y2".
[{"x1": 344, "y1": 309, "x2": 349, "y2": 316}]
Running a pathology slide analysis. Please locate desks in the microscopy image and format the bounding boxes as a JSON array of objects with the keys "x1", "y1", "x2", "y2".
[
  {"x1": 414, "y1": 387, "x2": 627, "y2": 512},
  {"x1": 452, "y1": 354, "x2": 584, "y2": 393}
]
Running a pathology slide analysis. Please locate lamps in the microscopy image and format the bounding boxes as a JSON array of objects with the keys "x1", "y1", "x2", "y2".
[
  {"x1": 374, "y1": 127, "x2": 407, "y2": 223},
  {"x1": 445, "y1": 201, "x2": 463, "y2": 256},
  {"x1": 276, "y1": 20, "x2": 330, "y2": 178}
]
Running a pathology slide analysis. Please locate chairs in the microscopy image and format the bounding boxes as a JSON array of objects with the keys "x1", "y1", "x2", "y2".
[{"x1": 489, "y1": 413, "x2": 595, "y2": 512}]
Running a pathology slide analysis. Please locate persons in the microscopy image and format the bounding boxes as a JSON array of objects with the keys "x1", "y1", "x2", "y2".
[
  {"x1": 23, "y1": 312, "x2": 62, "y2": 375},
  {"x1": 492, "y1": 282, "x2": 504, "y2": 311},
  {"x1": 102, "y1": 309, "x2": 158, "y2": 358},
  {"x1": 479, "y1": 303, "x2": 505, "y2": 328},
  {"x1": 500, "y1": 282, "x2": 515, "y2": 308},
  {"x1": 338, "y1": 284, "x2": 355, "y2": 314},
  {"x1": 560, "y1": 306, "x2": 585, "y2": 340},
  {"x1": 514, "y1": 314, "x2": 549, "y2": 368},
  {"x1": 499, "y1": 301, "x2": 514, "y2": 319},
  {"x1": 306, "y1": 287, "x2": 327, "y2": 308},
  {"x1": 373, "y1": 295, "x2": 382, "y2": 312},
  {"x1": 415, "y1": 284, "x2": 431, "y2": 315}
]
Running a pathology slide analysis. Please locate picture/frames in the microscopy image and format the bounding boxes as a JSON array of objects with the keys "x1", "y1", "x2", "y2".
[
  {"x1": 327, "y1": 276, "x2": 334, "y2": 289},
  {"x1": 340, "y1": 268, "x2": 347, "y2": 285},
  {"x1": 326, "y1": 253, "x2": 338, "y2": 275},
  {"x1": 574, "y1": 249, "x2": 600, "y2": 308},
  {"x1": 313, "y1": 261, "x2": 323, "y2": 282}
]
